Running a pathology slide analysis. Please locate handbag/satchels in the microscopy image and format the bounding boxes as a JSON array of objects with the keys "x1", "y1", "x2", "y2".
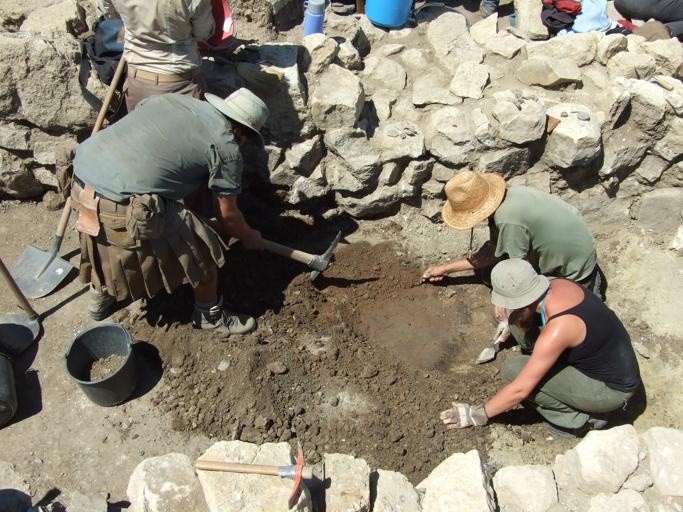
[{"x1": 86, "y1": 17, "x2": 127, "y2": 85}]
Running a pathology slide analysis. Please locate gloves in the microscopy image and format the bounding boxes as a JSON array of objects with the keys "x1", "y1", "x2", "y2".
[
  {"x1": 494, "y1": 319, "x2": 511, "y2": 345},
  {"x1": 440, "y1": 402, "x2": 487, "y2": 429}
]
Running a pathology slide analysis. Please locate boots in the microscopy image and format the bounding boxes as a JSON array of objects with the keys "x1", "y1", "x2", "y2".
[
  {"x1": 192, "y1": 292, "x2": 256, "y2": 336},
  {"x1": 89, "y1": 283, "x2": 115, "y2": 321}
]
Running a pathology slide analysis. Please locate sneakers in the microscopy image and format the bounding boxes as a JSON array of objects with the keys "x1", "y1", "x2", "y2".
[
  {"x1": 587, "y1": 269, "x2": 603, "y2": 302},
  {"x1": 548, "y1": 413, "x2": 608, "y2": 439},
  {"x1": 480, "y1": 0, "x2": 496, "y2": 18}
]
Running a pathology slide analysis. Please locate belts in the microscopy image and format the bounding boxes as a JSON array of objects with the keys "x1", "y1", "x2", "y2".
[
  {"x1": 128, "y1": 66, "x2": 200, "y2": 82},
  {"x1": 73, "y1": 173, "x2": 106, "y2": 199}
]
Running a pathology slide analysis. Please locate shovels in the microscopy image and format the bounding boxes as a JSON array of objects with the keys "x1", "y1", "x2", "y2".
[
  {"x1": 475, "y1": 329, "x2": 511, "y2": 365},
  {"x1": 11, "y1": 49, "x2": 129, "y2": 299}
]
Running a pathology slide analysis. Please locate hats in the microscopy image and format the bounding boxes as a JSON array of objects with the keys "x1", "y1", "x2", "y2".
[
  {"x1": 490, "y1": 259, "x2": 549, "y2": 309},
  {"x1": 442, "y1": 172, "x2": 506, "y2": 229},
  {"x1": 204, "y1": 88, "x2": 269, "y2": 149}
]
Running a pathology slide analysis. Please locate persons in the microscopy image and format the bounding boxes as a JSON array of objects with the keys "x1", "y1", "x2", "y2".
[
  {"x1": 479, "y1": 0, "x2": 516, "y2": 27},
  {"x1": 438, "y1": 258, "x2": 641, "y2": 438},
  {"x1": 68, "y1": 87, "x2": 270, "y2": 335},
  {"x1": 98, "y1": 0, "x2": 216, "y2": 114},
  {"x1": 422, "y1": 170, "x2": 597, "y2": 323}
]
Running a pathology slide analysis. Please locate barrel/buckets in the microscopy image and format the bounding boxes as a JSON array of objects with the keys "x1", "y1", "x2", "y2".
[
  {"x1": 0, "y1": 354, "x2": 19, "y2": 427},
  {"x1": 64, "y1": 322, "x2": 140, "y2": 407},
  {"x1": 364, "y1": 0, "x2": 413, "y2": 28}
]
